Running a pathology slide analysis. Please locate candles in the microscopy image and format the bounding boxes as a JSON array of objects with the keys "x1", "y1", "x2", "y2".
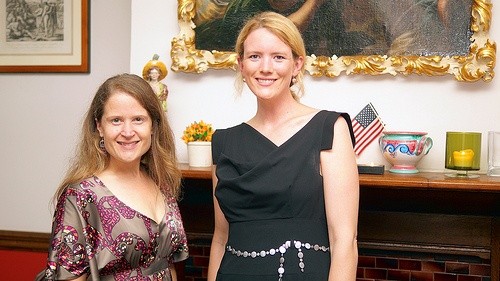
[{"x1": 453, "y1": 149, "x2": 474, "y2": 167}]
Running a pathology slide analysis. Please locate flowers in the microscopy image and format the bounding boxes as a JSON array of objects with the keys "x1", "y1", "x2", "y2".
[{"x1": 181, "y1": 120, "x2": 214, "y2": 143}]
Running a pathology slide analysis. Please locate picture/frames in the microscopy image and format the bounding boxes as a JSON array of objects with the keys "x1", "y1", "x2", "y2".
[
  {"x1": 171, "y1": 0, "x2": 497, "y2": 82},
  {"x1": 0, "y1": 0, "x2": 91, "y2": 74}
]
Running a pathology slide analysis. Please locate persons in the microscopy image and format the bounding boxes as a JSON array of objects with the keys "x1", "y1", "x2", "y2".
[
  {"x1": 207, "y1": 11, "x2": 360, "y2": 281},
  {"x1": 45, "y1": 73, "x2": 189, "y2": 281},
  {"x1": 142, "y1": 59, "x2": 168, "y2": 112}
]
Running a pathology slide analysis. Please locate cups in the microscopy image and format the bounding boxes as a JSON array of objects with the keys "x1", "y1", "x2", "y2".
[{"x1": 487, "y1": 131, "x2": 500, "y2": 177}]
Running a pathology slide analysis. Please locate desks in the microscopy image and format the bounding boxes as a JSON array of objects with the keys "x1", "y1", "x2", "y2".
[{"x1": 176, "y1": 162, "x2": 500, "y2": 281}]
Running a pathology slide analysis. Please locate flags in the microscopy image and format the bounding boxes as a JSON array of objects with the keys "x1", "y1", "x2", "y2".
[{"x1": 352, "y1": 101, "x2": 385, "y2": 156}]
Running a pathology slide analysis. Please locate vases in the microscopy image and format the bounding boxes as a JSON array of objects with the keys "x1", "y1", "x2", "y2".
[{"x1": 188, "y1": 141, "x2": 212, "y2": 171}]
